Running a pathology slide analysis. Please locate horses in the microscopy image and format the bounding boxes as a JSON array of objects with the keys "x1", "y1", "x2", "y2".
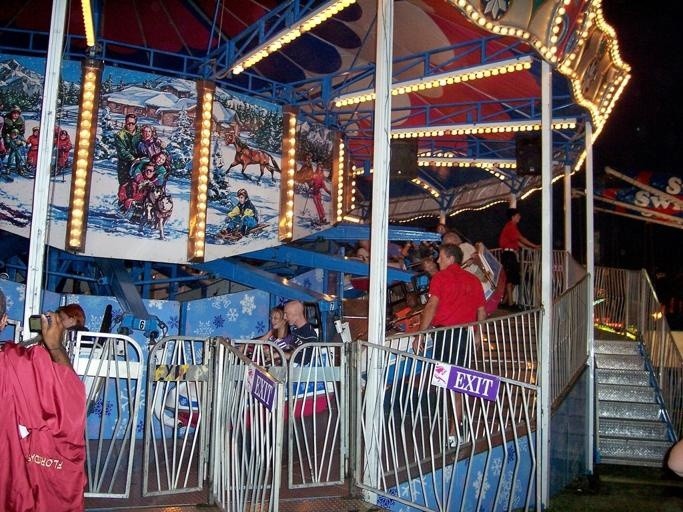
[{"x1": 224, "y1": 132, "x2": 281, "y2": 184}]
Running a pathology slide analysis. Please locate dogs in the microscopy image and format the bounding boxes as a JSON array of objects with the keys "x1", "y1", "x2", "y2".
[{"x1": 142, "y1": 185, "x2": 175, "y2": 240}]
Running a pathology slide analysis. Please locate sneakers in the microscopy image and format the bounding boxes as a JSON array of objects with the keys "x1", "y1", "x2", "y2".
[{"x1": 446, "y1": 436, "x2": 468, "y2": 450}]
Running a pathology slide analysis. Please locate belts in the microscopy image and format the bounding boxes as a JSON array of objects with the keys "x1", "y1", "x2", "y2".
[{"x1": 503, "y1": 246, "x2": 520, "y2": 264}]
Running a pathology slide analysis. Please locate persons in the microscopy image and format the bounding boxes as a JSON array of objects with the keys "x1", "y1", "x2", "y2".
[
  {"x1": 441, "y1": 230, "x2": 506, "y2": 349},
  {"x1": 497, "y1": 207, "x2": 542, "y2": 312},
  {"x1": 0, "y1": 104, "x2": 72, "y2": 178},
  {"x1": 111, "y1": 113, "x2": 169, "y2": 210},
  {"x1": 411, "y1": 244, "x2": 487, "y2": 450},
  {"x1": 263, "y1": 299, "x2": 318, "y2": 366},
  {"x1": 220, "y1": 187, "x2": 257, "y2": 238},
  {"x1": 0, "y1": 290, "x2": 85, "y2": 511},
  {"x1": 56, "y1": 303, "x2": 93, "y2": 348},
  {"x1": 300, "y1": 161, "x2": 332, "y2": 227},
  {"x1": 238, "y1": 306, "x2": 292, "y2": 366}
]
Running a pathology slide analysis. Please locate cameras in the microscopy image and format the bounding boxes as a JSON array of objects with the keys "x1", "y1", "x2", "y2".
[{"x1": 29, "y1": 315, "x2": 51, "y2": 333}]
